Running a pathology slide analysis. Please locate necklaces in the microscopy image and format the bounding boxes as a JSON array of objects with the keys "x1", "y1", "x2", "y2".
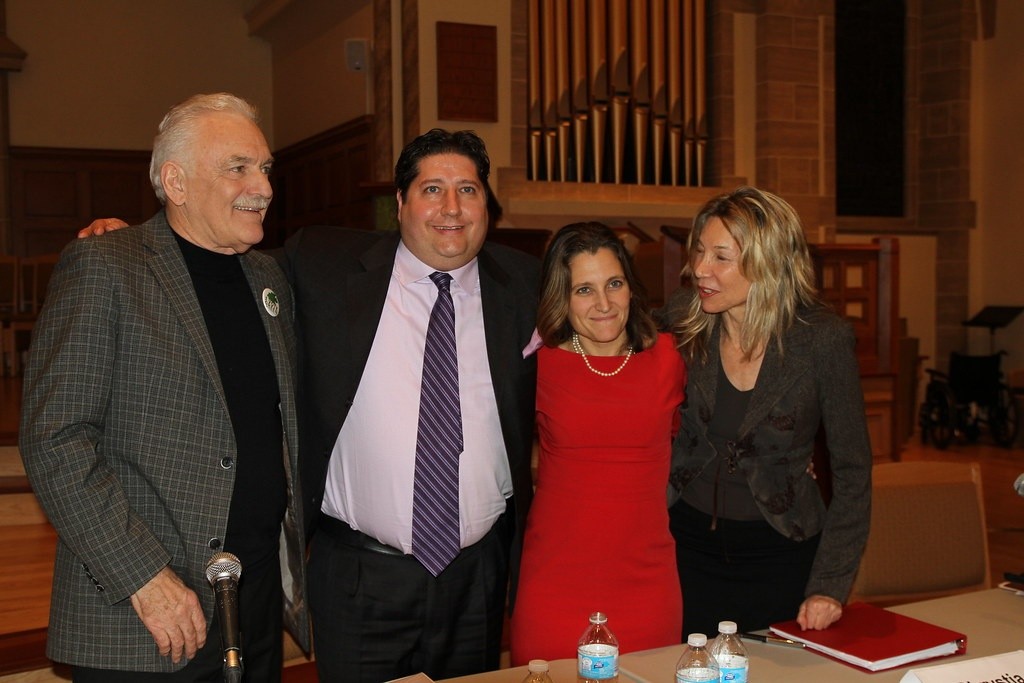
[{"x1": 572, "y1": 332, "x2": 634, "y2": 377}]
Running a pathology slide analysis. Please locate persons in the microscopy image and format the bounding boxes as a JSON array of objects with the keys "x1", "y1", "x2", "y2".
[
  {"x1": 511, "y1": 221, "x2": 817, "y2": 668},
  {"x1": 78, "y1": 128, "x2": 531, "y2": 683},
  {"x1": 669, "y1": 186, "x2": 873, "y2": 642},
  {"x1": 18, "y1": 92, "x2": 314, "y2": 683}
]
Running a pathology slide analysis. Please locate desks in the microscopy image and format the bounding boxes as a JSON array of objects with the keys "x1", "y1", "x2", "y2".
[{"x1": 434, "y1": 587, "x2": 1024, "y2": 683}]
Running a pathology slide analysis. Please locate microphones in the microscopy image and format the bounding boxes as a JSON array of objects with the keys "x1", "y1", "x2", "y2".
[
  {"x1": 204, "y1": 552, "x2": 243, "y2": 683},
  {"x1": 1013, "y1": 473, "x2": 1024, "y2": 496}
]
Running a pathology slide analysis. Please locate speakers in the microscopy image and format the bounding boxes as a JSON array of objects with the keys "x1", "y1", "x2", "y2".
[{"x1": 346, "y1": 37, "x2": 368, "y2": 71}]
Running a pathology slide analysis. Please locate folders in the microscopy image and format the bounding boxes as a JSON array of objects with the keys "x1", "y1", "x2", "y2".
[{"x1": 767, "y1": 602, "x2": 966, "y2": 674}]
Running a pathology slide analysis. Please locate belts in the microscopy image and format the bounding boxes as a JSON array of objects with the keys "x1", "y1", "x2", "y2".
[{"x1": 320, "y1": 513, "x2": 410, "y2": 559}]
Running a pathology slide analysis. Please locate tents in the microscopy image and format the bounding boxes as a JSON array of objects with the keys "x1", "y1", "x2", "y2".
[{"x1": 768, "y1": 600, "x2": 967, "y2": 674}]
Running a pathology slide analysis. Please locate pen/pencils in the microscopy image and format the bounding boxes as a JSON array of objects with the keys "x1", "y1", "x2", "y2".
[{"x1": 738, "y1": 633, "x2": 806, "y2": 648}]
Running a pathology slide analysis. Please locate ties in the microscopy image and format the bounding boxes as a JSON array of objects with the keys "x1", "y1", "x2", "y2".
[{"x1": 412, "y1": 271, "x2": 464, "y2": 579}]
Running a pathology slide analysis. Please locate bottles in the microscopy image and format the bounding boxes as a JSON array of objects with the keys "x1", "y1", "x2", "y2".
[
  {"x1": 577, "y1": 612, "x2": 619, "y2": 683},
  {"x1": 709, "y1": 621, "x2": 750, "y2": 683},
  {"x1": 677, "y1": 633, "x2": 719, "y2": 683},
  {"x1": 523, "y1": 660, "x2": 554, "y2": 683}
]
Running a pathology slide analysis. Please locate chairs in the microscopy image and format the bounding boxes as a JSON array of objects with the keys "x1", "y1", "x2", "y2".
[
  {"x1": 0, "y1": 255, "x2": 52, "y2": 378},
  {"x1": 845, "y1": 460, "x2": 992, "y2": 606}
]
